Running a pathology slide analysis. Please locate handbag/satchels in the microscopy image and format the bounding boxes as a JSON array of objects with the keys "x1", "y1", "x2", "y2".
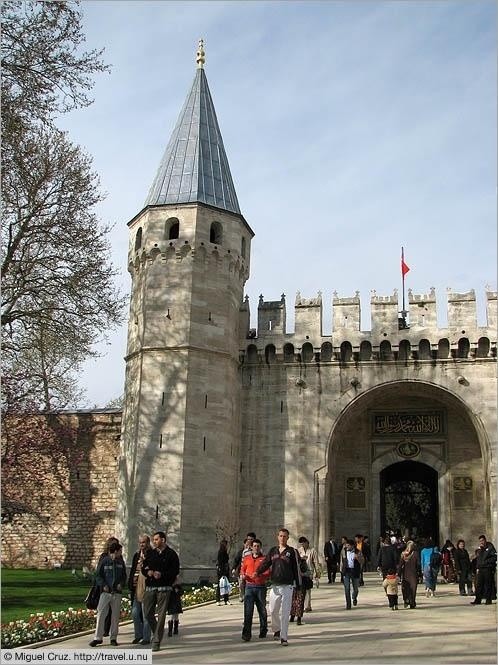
[
  {"x1": 295, "y1": 575, "x2": 312, "y2": 591},
  {"x1": 220, "y1": 575, "x2": 231, "y2": 596},
  {"x1": 85, "y1": 586, "x2": 100, "y2": 610}
]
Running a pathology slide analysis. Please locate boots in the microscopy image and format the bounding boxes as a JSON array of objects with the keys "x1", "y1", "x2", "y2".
[{"x1": 167, "y1": 620, "x2": 179, "y2": 637}]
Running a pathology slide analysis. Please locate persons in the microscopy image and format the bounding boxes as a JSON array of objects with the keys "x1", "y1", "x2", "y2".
[
  {"x1": 250, "y1": 529, "x2": 303, "y2": 647},
  {"x1": 215, "y1": 529, "x2": 496, "y2": 626},
  {"x1": 240, "y1": 539, "x2": 272, "y2": 642},
  {"x1": 88, "y1": 531, "x2": 184, "y2": 653}
]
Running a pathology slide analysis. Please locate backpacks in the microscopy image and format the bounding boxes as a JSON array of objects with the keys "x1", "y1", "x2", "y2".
[{"x1": 430, "y1": 547, "x2": 441, "y2": 568}]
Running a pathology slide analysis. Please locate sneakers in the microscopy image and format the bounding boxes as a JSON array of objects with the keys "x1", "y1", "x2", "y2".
[
  {"x1": 109, "y1": 639, "x2": 118, "y2": 646},
  {"x1": 132, "y1": 637, "x2": 150, "y2": 644},
  {"x1": 426, "y1": 588, "x2": 435, "y2": 597},
  {"x1": 460, "y1": 591, "x2": 467, "y2": 596},
  {"x1": 304, "y1": 608, "x2": 312, "y2": 613},
  {"x1": 346, "y1": 602, "x2": 351, "y2": 610},
  {"x1": 353, "y1": 593, "x2": 357, "y2": 606},
  {"x1": 391, "y1": 602, "x2": 398, "y2": 611},
  {"x1": 470, "y1": 600, "x2": 482, "y2": 605},
  {"x1": 327, "y1": 578, "x2": 344, "y2": 584},
  {"x1": 403, "y1": 600, "x2": 416, "y2": 609},
  {"x1": 468, "y1": 588, "x2": 475, "y2": 596},
  {"x1": 297, "y1": 616, "x2": 301, "y2": 625},
  {"x1": 152, "y1": 642, "x2": 160, "y2": 652},
  {"x1": 484, "y1": 599, "x2": 492, "y2": 605},
  {"x1": 88, "y1": 639, "x2": 103, "y2": 646},
  {"x1": 290, "y1": 614, "x2": 296, "y2": 621},
  {"x1": 273, "y1": 630, "x2": 280, "y2": 641},
  {"x1": 440, "y1": 578, "x2": 458, "y2": 585},
  {"x1": 259, "y1": 629, "x2": 267, "y2": 638},
  {"x1": 242, "y1": 634, "x2": 250, "y2": 641},
  {"x1": 280, "y1": 635, "x2": 289, "y2": 646},
  {"x1": 216, "y1": 602, "x2": 220, "y2": 606},
  {"x1": 226, "y1": 600, "x2": 233, "y2": 605}
]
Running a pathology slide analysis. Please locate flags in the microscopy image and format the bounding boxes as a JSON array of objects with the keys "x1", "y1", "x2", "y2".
[{"x1": 400, "y1": 260, "x2": 409, "y2": 275}]
